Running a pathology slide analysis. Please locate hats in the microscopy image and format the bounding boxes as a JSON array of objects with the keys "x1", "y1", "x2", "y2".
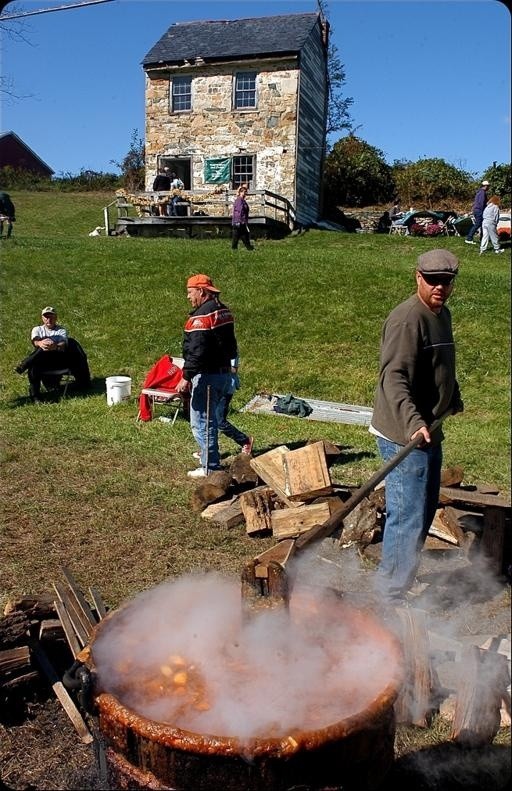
[
  {"x1": 41, "y1": 306, "x2": 56, "y2": 315},
  {"x1": 417, "y1": 249, "x2": 461, "y2": 275},
  {"x1": 481, "y1": 180, "x2": 491, "y2": 185},
  {"x1": 186, "y1": 273, "x2": 222, "y2": 294}
]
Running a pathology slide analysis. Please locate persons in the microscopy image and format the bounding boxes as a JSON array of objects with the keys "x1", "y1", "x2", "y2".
[
  {"x1": 377, "y1": 212, "x2": 392, "y2": 233},
  {"x1": 388, "y1": 198, "x2": 402, "y2": 221},
  {"x1": 464, "y1": 181, "x2": 490, "y2": 245},
  {"x1": 153, "y1": 168, "x2": 171, "y2": 216},
  {"x1": 168, "y1": 172, "x2": 185, "y2": 216},
  {"x1": 14, "y1": 306, "x2": 68, "y2": 405},
  {"x1": 0, "y1": 192, "x2": 16, "y2": 239},
  {"x1": 368, "y1": 248, "x2": 464, "y2": 618},
  {"x1": 479, "y1": 195, "x2": 504, "y2": 257},
  {"x1": 193, "y1": 349, "x2": 254, "y2": 456},
  {"x1": 231, "y1": 187, "x2": 256, "y2": 251},
  {"x1": 175, "y1": 273, "x2": 237, "y2": 479}
]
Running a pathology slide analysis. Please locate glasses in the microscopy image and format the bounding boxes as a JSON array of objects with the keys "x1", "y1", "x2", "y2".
[{"x1": 419, "y1": 271, "x2": 457, "y2": 286}]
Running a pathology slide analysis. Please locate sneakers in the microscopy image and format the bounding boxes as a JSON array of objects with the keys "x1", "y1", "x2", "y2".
[
  {"x1": 187, "y1": 467, "x2": 214, "y2": 478},
  {"x1": 15, "y1": 366, "x2": 24, "y2": 375},
  {"x1": 33, "y1": 395, "x2": 43, "y2": 403},
  {"x1": 193, "y1": 451, "x2": 202, "y2": 458},
  {"x1": 241, "y1": 436, "x2": 254, "y2": 455},
  {"x1": 495, "y1": 248, "x2": 505, "y2": 254},
  {"x1": 464, "y1": 239, "x2": 477, "y2": 245}
]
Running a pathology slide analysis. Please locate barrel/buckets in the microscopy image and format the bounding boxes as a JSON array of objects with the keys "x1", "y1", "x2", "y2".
[{"x1": 105, "y1": 374, "x2": 131, "y2": 408}]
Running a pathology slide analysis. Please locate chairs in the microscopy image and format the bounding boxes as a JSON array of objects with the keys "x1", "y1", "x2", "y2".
[
  {"x1": 388, "y1": 217, "x2": 408, "y2": 235},
  {"x1": 40, "y1": 339, "x2": 82, "y2": 398},
  {"x1": 136, "y1": 355, "x2": 187, "y2": 426}
]
